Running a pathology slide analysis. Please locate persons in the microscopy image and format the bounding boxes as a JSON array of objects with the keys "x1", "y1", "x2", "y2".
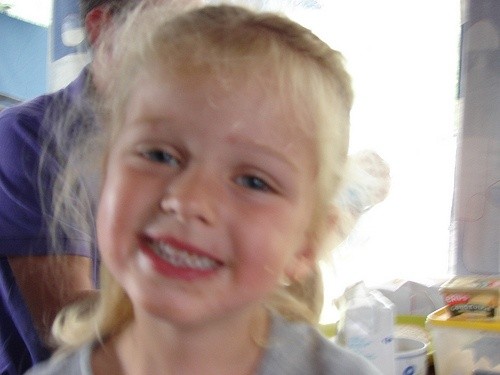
[
  {"x1": 0, "y1": 0, "x2": 215, "y2": 375},
  {"x1": 25, "y1": 0, "x2": 385, "y2": 375}
]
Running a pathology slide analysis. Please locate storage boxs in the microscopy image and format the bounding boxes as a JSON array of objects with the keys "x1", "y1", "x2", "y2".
[
  {"x1": 425, "y1": 305, "x2": 500, "y2": 375},
  {"x1": 438, "y1": 273, "x2": 500, "y2": 314}
]
